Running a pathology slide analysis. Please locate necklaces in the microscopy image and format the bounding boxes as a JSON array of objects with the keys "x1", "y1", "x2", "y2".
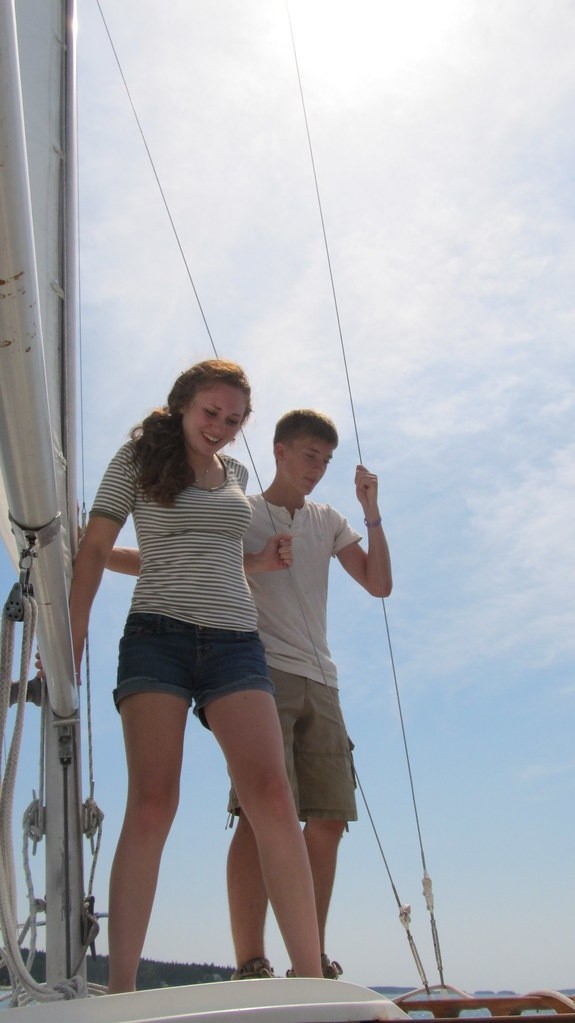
[{"x1": 194, "y1": 457, "x2": 213, "y2": 483}]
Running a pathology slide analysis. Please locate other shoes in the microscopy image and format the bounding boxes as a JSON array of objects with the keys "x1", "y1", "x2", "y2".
[
  {"x1": 230, "y1": 958, "x2": 276, "y2": 980},
  {"x1": 286, "y1": 954, "x2": 343, "y2": 980}
]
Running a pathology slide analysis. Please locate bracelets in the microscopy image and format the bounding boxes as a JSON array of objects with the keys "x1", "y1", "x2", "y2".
[{"x1": 363, "y1": 516, "x2": 381, "y2": 528}]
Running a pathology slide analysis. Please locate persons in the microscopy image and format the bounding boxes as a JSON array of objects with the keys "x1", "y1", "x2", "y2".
[
  {"x1": 71, "y1": 409, "x2": 393, "y2": 983},
  {"x1": 36, "y1": 359, "x2": 323, "y2": 994}
]
